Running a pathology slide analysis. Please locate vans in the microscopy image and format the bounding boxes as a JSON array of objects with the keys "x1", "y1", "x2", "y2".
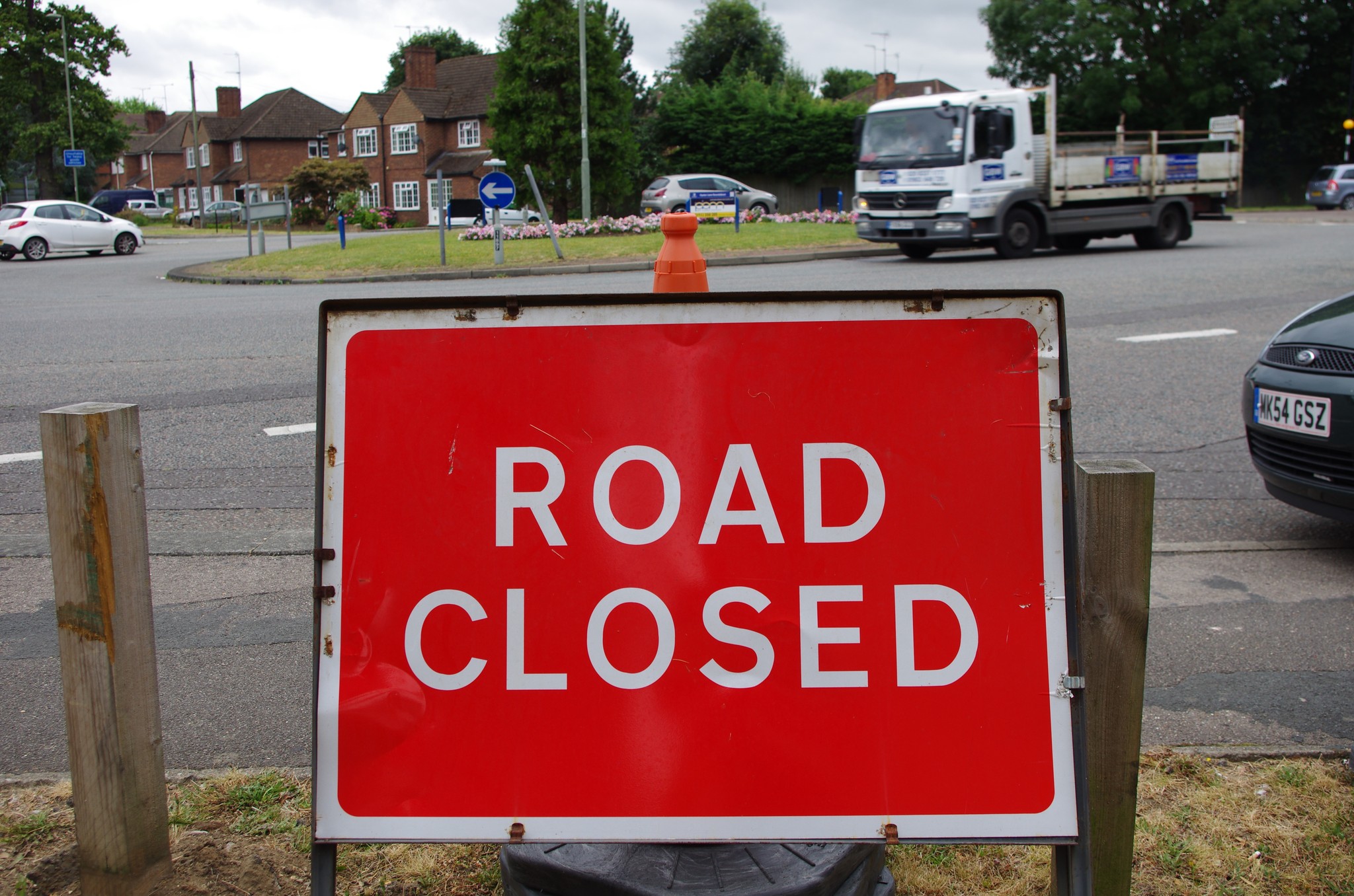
[{"x1": 88, "y1": 189, "x2": 157, "y2": 216}]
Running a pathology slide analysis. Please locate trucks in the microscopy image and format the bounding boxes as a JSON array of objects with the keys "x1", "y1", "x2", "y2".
[{"x1": 850, "y1": 74, "x2": 1243, "y2": 259}]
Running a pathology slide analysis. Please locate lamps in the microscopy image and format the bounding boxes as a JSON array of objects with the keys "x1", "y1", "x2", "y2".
[
  {"x1": 187, "y1": 179, "x2": 196, "y2": 187},
  {"x1": 337, "y1": 143, "x2": 349, "y2": 161},
  {"x1": 411, "y1": 135, "x2": 427, "y2": 172}
]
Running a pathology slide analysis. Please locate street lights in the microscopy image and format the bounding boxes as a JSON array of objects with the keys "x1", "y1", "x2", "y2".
[
  {"x1": 1342, "y1": 119, "x2": 1354, "y2": 164},
  {"x1": 46, "y1": 13, "x2": 79, "y2": 203}
]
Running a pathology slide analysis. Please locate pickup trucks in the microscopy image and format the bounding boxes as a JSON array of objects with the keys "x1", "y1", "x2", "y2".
[{"x1": 444, "y1": 198, "x2": 542, "y2": 228}]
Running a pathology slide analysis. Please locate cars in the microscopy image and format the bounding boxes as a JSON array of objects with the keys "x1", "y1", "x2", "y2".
[
  {"x1": 124, "y1": 200, "x2": 179, "y2": 219},
  {"x1": 0, "y1": 200, "x2": 147, "y2": 260},
  {"x1": 1304, "y1": 165, "x2": 1354, "y2": 211},
  {"x1": 176, "y1": 201, "x2": 245, "y2": 227},
  {"x1": 641, "y1": 173, "x2": 780, "y2": 216},
  {"x1": 1240, "y1": 290, "x2": 1354, "y2": 520}
]
